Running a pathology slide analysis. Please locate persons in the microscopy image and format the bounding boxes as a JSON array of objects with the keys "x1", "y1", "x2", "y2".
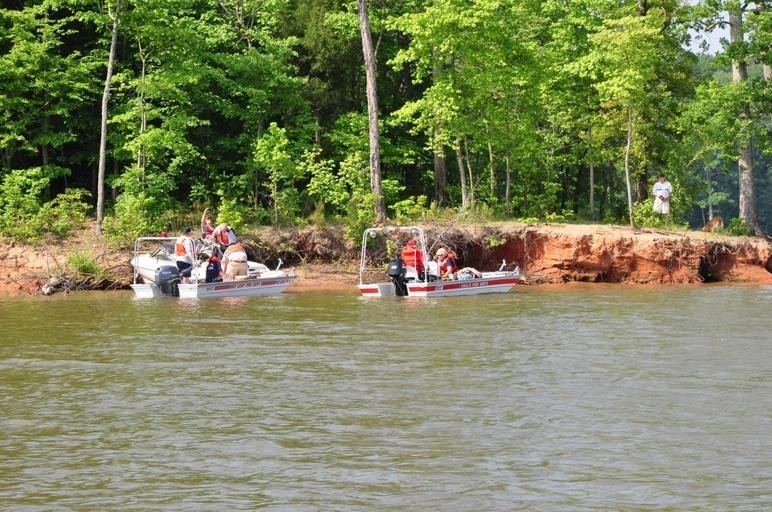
[
  {"x1": 651, "y1": 173, "x2": 673, "y2": 228},
  {"x1": 173, "y1": 227, "x2": 199, "y2": 283},
  {"x1": 433, "y1": 247, "x2": 462, "y2": 283},
  {"x1": 401, "y1": 239, "x2": 430, "y2": 280},
  {"x1": 200, "y1": 204, "x2": 249, "y2": 284}
]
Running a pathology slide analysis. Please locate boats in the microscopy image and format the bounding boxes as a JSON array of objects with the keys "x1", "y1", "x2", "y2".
[
  {"x1": 356, "y1": 226, "x2": 524, "y2": 298},
  {"x1": 129, "y1": 236, "x2": 299, "y2": 303}
]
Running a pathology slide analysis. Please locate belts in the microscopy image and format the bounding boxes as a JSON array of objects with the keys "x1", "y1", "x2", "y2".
[{"x1": 230, "y1": 260, "x2": 247, "y2": 263}]
